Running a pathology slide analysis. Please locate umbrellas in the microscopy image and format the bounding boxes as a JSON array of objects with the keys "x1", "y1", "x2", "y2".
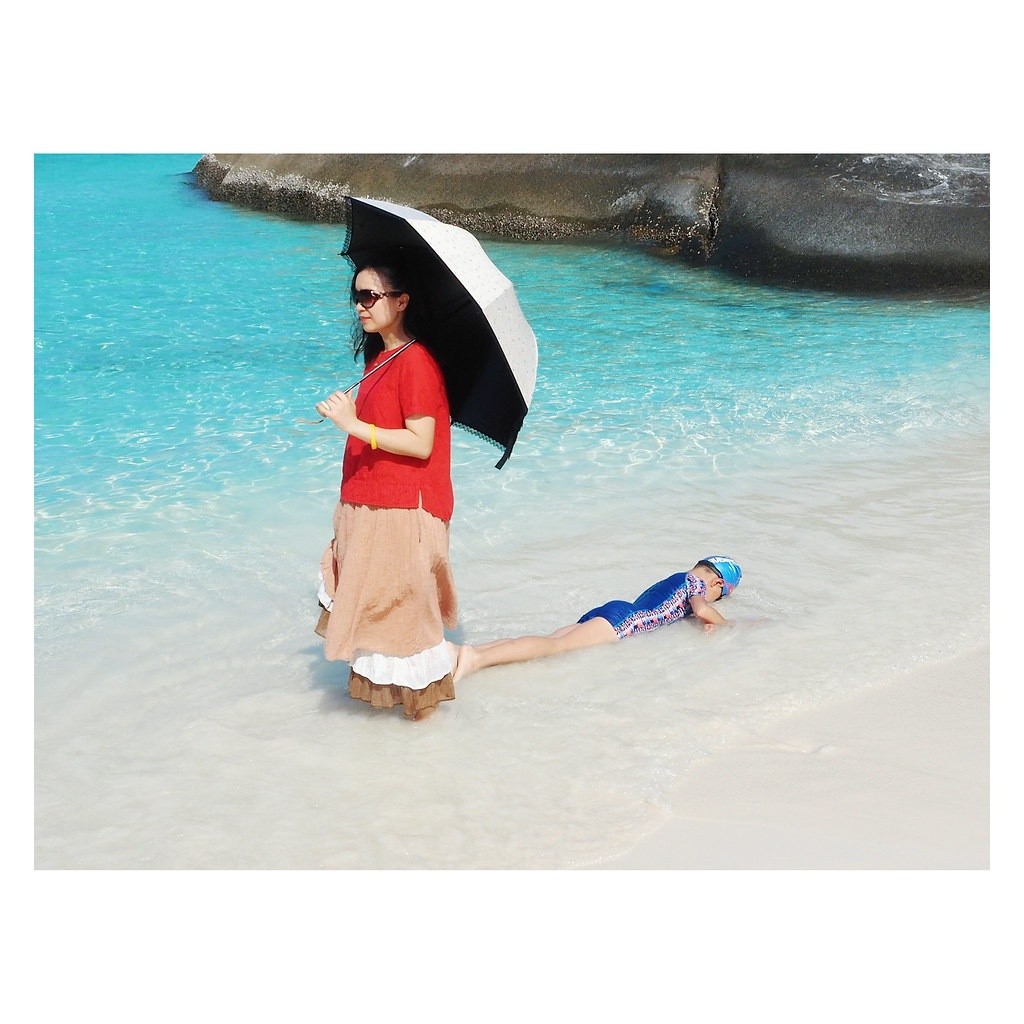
[{"x1": 315, "y1": 194, "x2": 538, "y2": 470}]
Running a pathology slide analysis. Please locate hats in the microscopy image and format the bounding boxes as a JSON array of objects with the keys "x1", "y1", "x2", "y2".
[{"x1": 700, "y1": 556, "x2": 742, "y2": 595}]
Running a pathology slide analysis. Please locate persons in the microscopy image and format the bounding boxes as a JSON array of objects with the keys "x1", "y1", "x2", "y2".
[
  {"x1": 452, "y1": 556, "x2": 741, "y2": 685},
  {"x1": 317, "y1": 261, "x2": 458, "y2": 723}
]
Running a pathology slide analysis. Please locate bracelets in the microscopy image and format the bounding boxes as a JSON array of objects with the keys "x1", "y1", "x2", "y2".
[{"x1": 370, "y1": 424, "x2": 377, "y2": 450}]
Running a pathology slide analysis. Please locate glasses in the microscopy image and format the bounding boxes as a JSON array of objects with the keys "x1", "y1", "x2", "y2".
[
  {"x1": 352, "y1": 289, "x2": 402, "y2": 308},
  {"x1": 715, "y1": 593, "x2": 723, "y2": 601}
]
